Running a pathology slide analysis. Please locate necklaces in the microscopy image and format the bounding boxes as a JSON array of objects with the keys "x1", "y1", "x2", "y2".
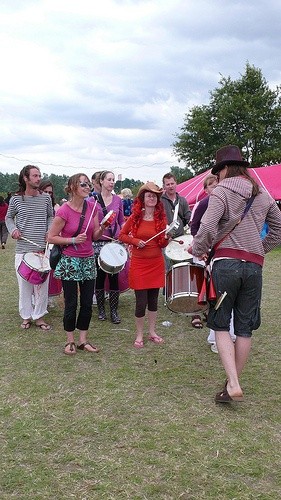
[
  {"x1": 144, "y1": 207, "x2": 154, "y2": 220},
  {"x1": 69, "y1": 200, "x2": 85, "y2": 211}
]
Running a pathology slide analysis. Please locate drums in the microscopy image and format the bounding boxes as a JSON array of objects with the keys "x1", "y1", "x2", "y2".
[
  {"x1": 98, "y1": 243, "x2": 127, "y2": 274},
  {"x1": 17, "y1": 252, "x2": 50, "y2": 286},
  {"x1": 165, "y1": 262, "x2": 210, "y2": 316},
  {"x1": 101, "y1": 210, "x2": 117, "y2": 227},
  {"x1": 165, "y1": 235, "x2": 194, "y2": 267},
  {"x1": 32, "y1": 269, "x2": 63, "y2": 297}
]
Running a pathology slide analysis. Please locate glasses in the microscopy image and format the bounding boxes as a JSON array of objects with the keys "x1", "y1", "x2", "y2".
[
  {"x1": 43, "y1": 191, "x2": 54, "y2": 194},
  {"x1": 214, "y1": 169, "x2": 223, "y2": 175},
  {"x1": 78, "y1": 182, "x2": 92, "y2": 187}
]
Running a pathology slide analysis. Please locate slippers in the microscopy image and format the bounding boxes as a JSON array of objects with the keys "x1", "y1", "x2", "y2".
[
  {"x1": 35, "y1": 322, "x2": 50, "y2": 330},
  {"x1": 134, "y1": 338, "x2": 145, "y2": 348},
  {"x1": 77, "y1": 341, "x2": 100, "y2": 353},
  {"x1": 20, "y1": 321, "x2": 31, "y2": 329},
  {"x1": 148, "y1": 334, "x2": 163, "y2": 343},
  {"x1": 65, "y1": 342, "x2": 76, "y2": 355},
  {"x1": 215, "y1": 378, "x2": 243, "y2": 402}
]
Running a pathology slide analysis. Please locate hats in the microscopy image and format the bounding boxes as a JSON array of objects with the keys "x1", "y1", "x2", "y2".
[
  {"x1": 137, "y1": 182, "x2": 163, "y2": 197},
  {"x1": 211, "y1": 145, "x2": 250, "y2": 175}
]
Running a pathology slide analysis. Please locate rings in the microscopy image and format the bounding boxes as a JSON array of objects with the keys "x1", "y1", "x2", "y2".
[{"x1": 80, "y1": 239, "x2": 81, "y2": 241}]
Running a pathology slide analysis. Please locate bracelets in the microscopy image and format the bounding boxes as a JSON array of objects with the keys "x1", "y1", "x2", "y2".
[
  {"x1": 71, "y1": 237, "x2": 75, "y2": 246},
  {"x1": 99, "y1": 227, "x2": 104, "y2": 232}
]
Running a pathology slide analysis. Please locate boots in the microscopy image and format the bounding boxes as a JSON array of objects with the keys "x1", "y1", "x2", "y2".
[
  {"x1": 109, "y1": 289, "x2": 121, "y2": 324},
  {"x1": 95, "y1": 289, "x2": 108, "y2": 320}
]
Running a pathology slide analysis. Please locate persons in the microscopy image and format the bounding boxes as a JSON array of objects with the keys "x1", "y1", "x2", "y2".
[
  {"x1": 85, "y1": 171, "x2": 133, "y2": 324},
  {"x1": 160, "y1": 173, "x2": 192, "y2": 295},
  {"x1": 192, "y1": 145, "x2": 281, "y2": 403},
  {"x1": 118, "y1": 182, "x2": 172, "y2": 348},
  {"x1": 0, "y1": 179, "x2": 69, "y2": 248},
  {"x1": 188, "y1": 178, "x2": 238, "y2": 354},
  {"x1": 5, "y1": 165, "x2": 55, "y2": 331},
  {"x1": 46, "y1": 173, "x2": 104, "y2": 355}
]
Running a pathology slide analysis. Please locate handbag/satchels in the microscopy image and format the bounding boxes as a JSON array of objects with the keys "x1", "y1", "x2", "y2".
[{"x1": 49, "y1": 243, "x2": 61, "y2": 270}]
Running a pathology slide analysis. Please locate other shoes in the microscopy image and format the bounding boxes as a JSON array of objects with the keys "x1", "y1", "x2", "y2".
[
  {"x1": 1, "y1": 244, "x2": 6, "y2": 250},
  {"x1": 211, "y1": 343, "x2": 220, "y2": 354}
]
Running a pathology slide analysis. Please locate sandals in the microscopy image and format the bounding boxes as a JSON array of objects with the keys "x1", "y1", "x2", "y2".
[{"x1": 190, "y1": 316, "x2": 203, "y2": 329}]
